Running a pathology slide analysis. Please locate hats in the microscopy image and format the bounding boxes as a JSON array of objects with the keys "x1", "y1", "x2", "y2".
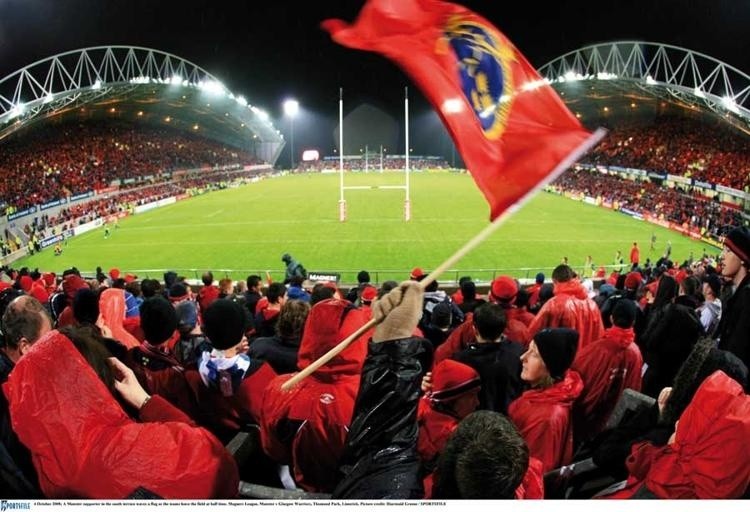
[
  {"x1": 431, "y1": 359, "x2": 481, "y2": 402},
  {"x1": 410, "y1": 268, "x2": 423, "y2": 278},
  {"x1": 724, "y1": 226, "x2": 749, "y2": 262},
  {"x1": 534, "y1": 328, "x2": 579, "y2": 374},
  {"x1": 361, "y1": 287, "x2": 378, "y2": 302},
  {"x1": 624, "y1": 272, "x2": 641, "y2": 288},
  {"x1": 489, "y1": 277, "x2": 519, "y2": 304}
]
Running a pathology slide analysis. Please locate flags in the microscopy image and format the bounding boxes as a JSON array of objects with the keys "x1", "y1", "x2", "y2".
[{"x1": 321, "y1": 0, "x2": 603, "y2": 215}]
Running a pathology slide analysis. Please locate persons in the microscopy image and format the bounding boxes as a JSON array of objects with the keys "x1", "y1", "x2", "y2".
[{"x1": 0, "y1": 119, "x2": 749, "y2": 500}]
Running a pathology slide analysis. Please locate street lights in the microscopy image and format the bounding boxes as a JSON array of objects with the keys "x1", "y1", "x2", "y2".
[{"x1": 283, "y1": 99, "x2": 301, "y2": 172}]
